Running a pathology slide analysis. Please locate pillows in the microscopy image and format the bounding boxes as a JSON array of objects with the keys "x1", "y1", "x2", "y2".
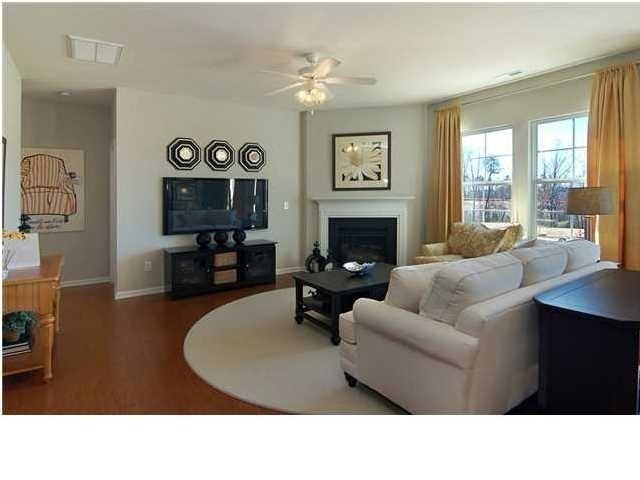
[
  {"x1": 460, "y1": 222, "x2": 508, "y2": 257},
  {"x1": 384, "y1": 262, "x2": 451, "y2": 314}
]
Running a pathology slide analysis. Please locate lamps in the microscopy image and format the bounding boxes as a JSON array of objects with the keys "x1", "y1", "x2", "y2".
[
  {"x1": 293, "y1": 82, "x2": 328, "y2": 116},
  {"x1": 567, "y1": 187, "x2": 617, "y2": 243}
]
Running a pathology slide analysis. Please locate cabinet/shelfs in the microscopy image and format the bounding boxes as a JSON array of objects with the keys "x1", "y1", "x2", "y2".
[{"x1": 162, "y1": 239, "x2": 279, "y2": 301}]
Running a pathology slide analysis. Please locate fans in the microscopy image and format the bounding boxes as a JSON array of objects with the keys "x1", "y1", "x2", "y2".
[{"x1": 259, "y1": 52, "x2": 377, "y2": 101}]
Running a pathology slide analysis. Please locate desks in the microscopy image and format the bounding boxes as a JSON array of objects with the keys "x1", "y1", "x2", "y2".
[
  {"x1": 2, "y1": 254, "x2": 65, "y2": 383},
  {"x1": 532, "y1": 268, "x2": 639, "y2": 414}
]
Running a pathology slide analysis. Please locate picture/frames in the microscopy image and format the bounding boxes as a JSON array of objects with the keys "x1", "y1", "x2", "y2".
[{"x1": 331, "y1": 131, "x2": 391, "y2": 191}]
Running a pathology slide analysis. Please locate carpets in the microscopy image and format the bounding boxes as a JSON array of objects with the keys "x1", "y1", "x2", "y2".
[{"x1": 182, "y1": 285, "x2": 407, "y2": 414}]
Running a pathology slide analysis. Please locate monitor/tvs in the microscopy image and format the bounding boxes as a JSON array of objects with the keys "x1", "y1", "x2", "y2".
[{"x1": 162, "y1": 176, "x2": 269, "y2": 234}]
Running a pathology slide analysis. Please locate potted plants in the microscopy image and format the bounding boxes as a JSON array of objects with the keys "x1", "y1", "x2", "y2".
[{"x1": 2, "y1": 311, "x2": 37, "y2": 343}]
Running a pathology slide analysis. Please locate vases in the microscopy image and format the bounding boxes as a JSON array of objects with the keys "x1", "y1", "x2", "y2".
[{"x1": 2, "y1": 270, "x2": 9, "y2": 281}]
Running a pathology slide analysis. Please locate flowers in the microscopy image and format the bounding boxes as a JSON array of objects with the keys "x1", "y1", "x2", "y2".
[{"x1": 2, "y1": 231, "x2": 25, "y2": 270}]
